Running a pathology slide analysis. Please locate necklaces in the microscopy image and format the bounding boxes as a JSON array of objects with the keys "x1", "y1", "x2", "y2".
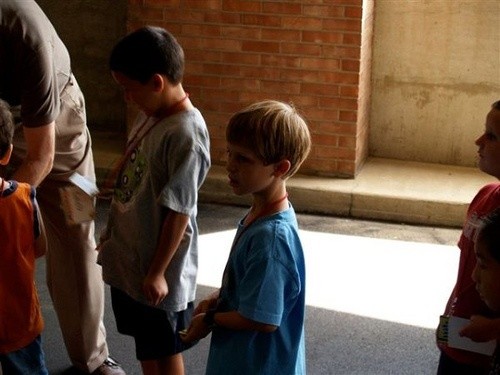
[
  {"x1": 121, "y1": 93, "x2": 190, "y2": 162},
  {"x1": 234, "y1": 192, "x2": 288, "y2": 246}
]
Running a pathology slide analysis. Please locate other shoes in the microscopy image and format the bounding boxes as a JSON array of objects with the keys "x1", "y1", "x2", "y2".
[{"x1": 95, "y1": 356, "x2": 127, "y2": 375}]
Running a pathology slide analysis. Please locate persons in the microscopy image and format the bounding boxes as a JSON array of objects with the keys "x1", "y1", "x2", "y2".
[
  {"x1": 0, "y1": 0, "x2": 125, "y2": 375},
  {"x1": 180, "y1": 101, "x2": 311, "y2": 375},
  {"x1": 92, "y1": 26, "x2": 210, "y2": 375},
  {"x1": 0, "y1": 99, "x2": 47, "y2": 375},
  {"x1": 435, "y1": 100, "x2": 500, "y2": 375}
]
